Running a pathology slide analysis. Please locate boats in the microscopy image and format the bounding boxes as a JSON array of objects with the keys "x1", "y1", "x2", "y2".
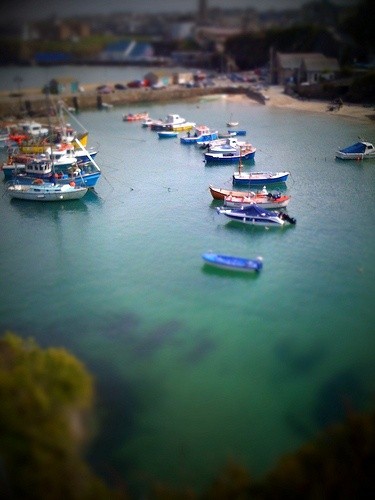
[
  {"x1": 208, "y1": 184, "x2": 292, "y2": 210},
  {"x1": 7, "y1": 161, "x2": 102, "y2": 191},
  {"x1": 7, "y1": 181, "x2": 89, "y2": 201},
  {"x1": 233, "y1": 169, "x2": 291, "y2": 186},
  {"x1": 121, "y1": 111, "x2": 149, "y2": 122},
  {"x1": 201, "y1": 251, "x2": 264, "y2": 274},
  {"x1": 335, "y1": 140, "x2": 375, "y2": 160},
  {"x1": 248, "y1": 86, "x2": 272, "y2": 102},
  {"x1": 1, "y1": 157, "x2": 79, "y2": 180},
  {"x1": 204, "y1": 143, "x2": 257, "y2": 163},
  {"x1": 156, "y1": 130, "x2": 178, "y2": 141},
  {"x1": 141, "y1": 113, "x2": 255, "y2": 150},
  {"x1": 215, "y1": 201, "x2": 297, "y2": 229},
  {"x1": 0, "y1": 117, "x2": 100, "y2": 164}
]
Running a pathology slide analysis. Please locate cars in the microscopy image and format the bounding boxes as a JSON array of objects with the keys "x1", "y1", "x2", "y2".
[
  {"x1": 97, "y1": 79, "x2": 142, "y2": 95},
  {"x1": 186, "y1": 70, "x2": 260, "y2": 89}
]
